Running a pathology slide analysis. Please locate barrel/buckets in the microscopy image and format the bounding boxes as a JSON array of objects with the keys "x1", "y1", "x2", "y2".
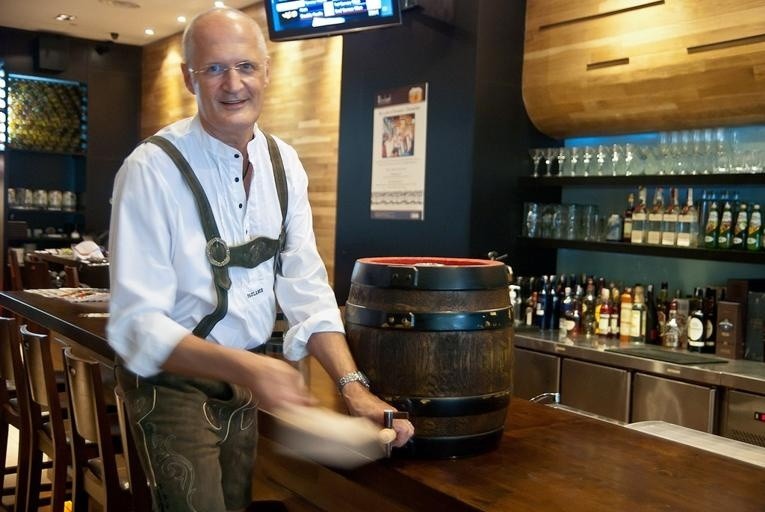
[{"x1": 344, "y1": 256, "x2": 516, "y2": 461}]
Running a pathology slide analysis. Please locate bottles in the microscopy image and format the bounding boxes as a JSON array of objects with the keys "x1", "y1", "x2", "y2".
[
  {"x1": 623, "y1": 191, "x2": 635, "y2": 240},
  {"x1": 512, "y1": 271, "x2": 717, "y2": 354},
  {"x1": 698, "y1": 189, "x2": 763, "y2": 253},
  {"x1": 629, "y1": 184, "x2": 699, "y2": 250}
]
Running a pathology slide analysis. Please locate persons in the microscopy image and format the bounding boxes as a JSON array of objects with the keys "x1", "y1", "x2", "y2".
[
  {"x1": 106, "y1": 7, "x2": 415, "y2": 512},
  {"x1": 380, "y1": 114, "x2": 414, "y2": 158}
]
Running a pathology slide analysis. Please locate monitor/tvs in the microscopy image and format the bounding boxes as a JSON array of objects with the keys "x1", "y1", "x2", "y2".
[{"x1": 261, "y1": 0, "x2": 404, "y2": 42}]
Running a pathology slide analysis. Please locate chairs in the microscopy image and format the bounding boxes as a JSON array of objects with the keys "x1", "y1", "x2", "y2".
[
  {"x1": 59, "y1": 346, "x2": 153, "y2": 512},
  {"x1": 17, "y1": 325, "x2": 123, "y2": 512},
  {"x1": 0, "y1": 317, "x2": 64, "y2": 511},
  {"x1": 111, "y1": 383, "x2": 287, "y2": 512}
]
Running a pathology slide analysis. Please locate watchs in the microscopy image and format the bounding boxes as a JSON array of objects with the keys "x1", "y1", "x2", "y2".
[{"x1": 335, "y1": 370, "x2": 374, "y2": 393}]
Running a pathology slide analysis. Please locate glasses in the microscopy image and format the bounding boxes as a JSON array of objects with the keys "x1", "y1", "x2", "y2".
[{"x1": 189, "y1": 59, "x2": 269, "y2": 82}]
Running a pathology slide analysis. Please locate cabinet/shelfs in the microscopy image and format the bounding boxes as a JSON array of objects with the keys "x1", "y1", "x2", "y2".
[
  {"x1": 505, "y1": 170, "x2": 764, "y2": 455},
  {"x1": 0, "y1": 66, "x2": 89, "y2": 253}
]
